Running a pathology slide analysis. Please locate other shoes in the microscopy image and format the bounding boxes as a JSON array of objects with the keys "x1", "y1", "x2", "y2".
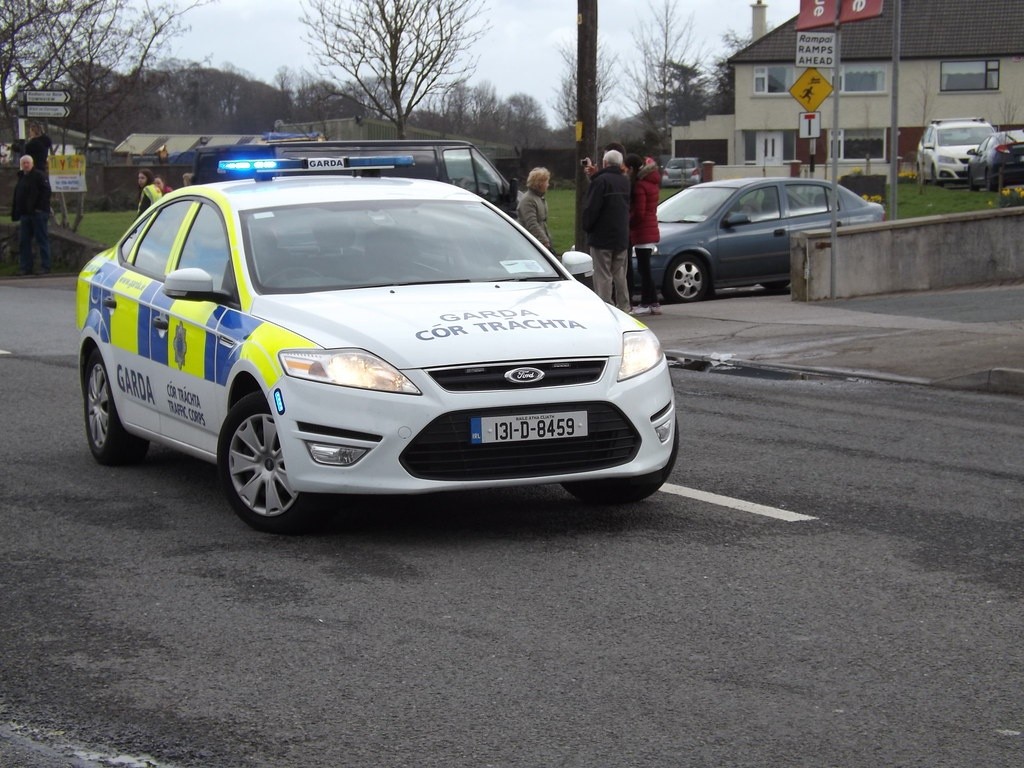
[
  {"x1": 15, "y1": 268, "x2": 33, "y2": 276},
  {"x1": 651, "y1": 303, "x2": 663, "y2": 314},
  {"x1": 628, "y1": 305, "x2": 651, "y2": 317},
  {"x1": 35, "y1": 267, "x2": 52, "y2": 275}
]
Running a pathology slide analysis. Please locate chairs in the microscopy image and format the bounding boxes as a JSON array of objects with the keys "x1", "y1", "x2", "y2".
[
  {"x1": 312, "y1": 216, "x2": 370, "y2": 285},
  {"x1": 222, "y1": 229, "x2": 291, "y2": 296}
]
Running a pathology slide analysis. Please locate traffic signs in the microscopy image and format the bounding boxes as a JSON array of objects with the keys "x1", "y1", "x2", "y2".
[{"x1": 19, "y1": 90, "x2": 70, "y2": 120}]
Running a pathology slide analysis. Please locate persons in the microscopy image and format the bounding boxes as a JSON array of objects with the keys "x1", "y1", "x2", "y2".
[
  {"x1": 135, "y1": 168, "x2": 196, "y2": 224},
  {"x1": 10, "y1": 122, "x2": 53, "y2": 275},
  {"x1": 580, "y1": 143, "x2": 661, "y2": 316},
  {"x1": 517, "y1": 167, "x2": 558, "y2": 257}
]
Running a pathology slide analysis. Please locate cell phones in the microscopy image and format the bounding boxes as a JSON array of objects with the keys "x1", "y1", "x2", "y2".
[{"x1": 581, "y1": 160, "x2": 587, "y2": 166}]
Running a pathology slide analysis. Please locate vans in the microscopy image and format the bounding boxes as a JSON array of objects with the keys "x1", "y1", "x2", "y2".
[{"x1": 188, "y1": 136, "x2": 518, "y2": 226}]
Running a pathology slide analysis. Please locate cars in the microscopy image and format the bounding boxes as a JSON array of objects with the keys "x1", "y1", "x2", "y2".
[
  {"x1": 564, "y1": 173, "x2": 891, "y2": 303},
  {"x1": 72, "y1": 149, "x2": 681, "y2": 536},
  {"x1": 917, "y1": 116, "x2": 1024, "y2": 192}
]
